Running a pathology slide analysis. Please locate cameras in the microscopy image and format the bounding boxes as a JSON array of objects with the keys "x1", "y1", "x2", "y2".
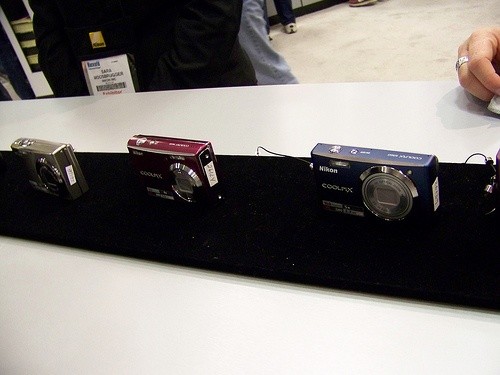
[
  {"x1": 11, "y1": 137, "x2": 88, "y2": 201},
  {"x1": 311, "y1": 142, "x2": 441, "y2": 222},
  {"x1": 127, "y1": 133, "x2": 223, "y2": 205}
]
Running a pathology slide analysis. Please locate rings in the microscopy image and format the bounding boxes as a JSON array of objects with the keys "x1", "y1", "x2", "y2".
[{"x1": 455, "y1": 56, "x2": 468, "y2": 70}]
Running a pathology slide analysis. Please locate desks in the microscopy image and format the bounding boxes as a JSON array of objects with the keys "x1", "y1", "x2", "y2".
[{"x1": 0, "y1": 86, "x2": 500, "y2": 375}]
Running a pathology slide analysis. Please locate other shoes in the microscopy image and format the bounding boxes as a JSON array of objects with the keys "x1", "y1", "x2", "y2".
[{"x1": 348, "y1": 0, "x2": 377, "y2": 7}]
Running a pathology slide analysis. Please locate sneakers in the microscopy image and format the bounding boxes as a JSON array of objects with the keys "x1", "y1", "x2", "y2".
[{"x1": 284, "y1": 22, "x2": 297, "y2": 34}]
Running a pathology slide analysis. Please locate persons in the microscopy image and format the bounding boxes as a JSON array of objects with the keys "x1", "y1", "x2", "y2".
[
  {"x1": 0, "y1": 16, "x2": 35, "y2": 101},
  {"x1": 33, "y1": 1, "x2": 256, "y2": 98},
  {"x1": 237, "y1": 0, "x2": 299, "y2": 85},
  {"x1": 263, "y1": 0, "x2": 298, "y2": 41},
  {"x1": 455, "y1": 26, "x2": 500, "y2": 102}
]
